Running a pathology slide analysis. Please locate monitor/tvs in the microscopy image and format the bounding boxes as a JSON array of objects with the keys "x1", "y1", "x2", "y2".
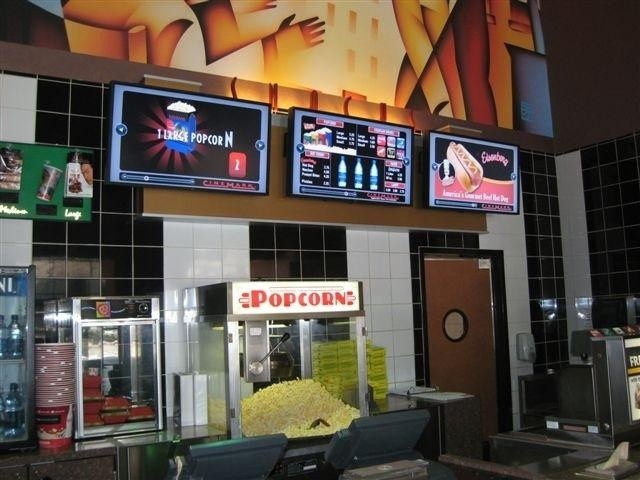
[
  {"x1": 421, "y1": 129, "x2": 520, "y2": 215},
  {"x1": 286, "y1": 106, "x2": 414, "y2": 207},
  {"x1": 104, "y1": 81, "x2": 272, "y2": 195},
  {"x1": 167, "y1": 433, "x2": 290, "y2": 480},
  {"x1": 324, "y1": 409, "x2": 431, "y2": 470},
  {"x1": 518, "y1": 366, "x2": 598, "y2": 431}
]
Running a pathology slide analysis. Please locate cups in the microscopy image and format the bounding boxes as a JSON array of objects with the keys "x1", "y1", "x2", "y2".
[
  {"x1": 36, "y1": 164, "x2": 62, "y2": 202},
  {"x1": 33, "y1": 342, "x2": 76, "y2": 451}
]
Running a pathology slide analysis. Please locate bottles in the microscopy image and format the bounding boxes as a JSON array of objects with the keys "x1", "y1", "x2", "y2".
[
  {"x1": 0, "y1": 313, "x2": 25, "y2": 440},
  {"x1": 339, "y1": 156, "x2": 381, "y2": 190}
]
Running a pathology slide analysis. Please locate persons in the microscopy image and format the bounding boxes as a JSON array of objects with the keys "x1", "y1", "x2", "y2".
[{"x1": 80, "y1": 153, "x2": 94, "y2": 186}]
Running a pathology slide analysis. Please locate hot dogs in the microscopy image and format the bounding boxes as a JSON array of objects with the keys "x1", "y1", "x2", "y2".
[{"x1": 446, "y1": 141, "x2": 483, "y2": 192}]
